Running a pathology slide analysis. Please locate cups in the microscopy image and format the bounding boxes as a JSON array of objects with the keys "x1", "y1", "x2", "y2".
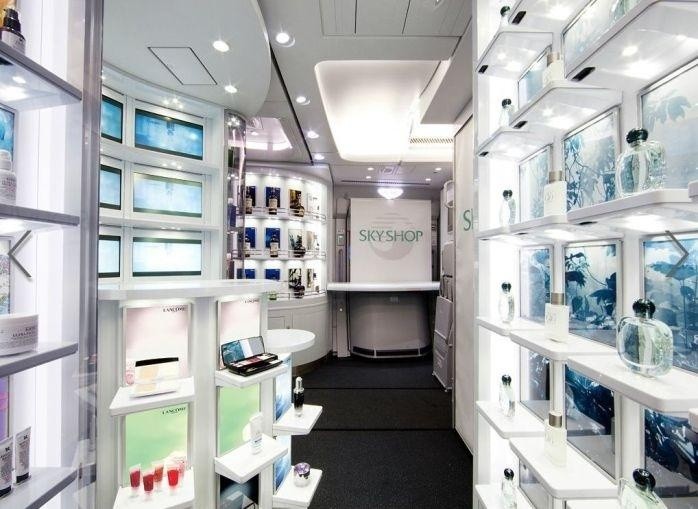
[{"x1": 293, "y1": 462, "x2": 311, "y2": 487}]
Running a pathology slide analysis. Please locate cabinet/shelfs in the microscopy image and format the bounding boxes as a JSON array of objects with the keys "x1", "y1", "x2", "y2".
[{"x1": 473, "y1": 1, "x2": 698, "y2": 509}]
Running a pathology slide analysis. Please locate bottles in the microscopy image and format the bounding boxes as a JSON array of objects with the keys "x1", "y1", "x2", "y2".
[
  {"x1": 610, "y1": 1, "x2": 640, "y2": 21},
  {"x1": 614, "y1": 127, "x2": 666, "y2": 199},
  {"x1": 500, "y1": 99, "x2": 516, "y2": 128},
  {"x1": 2, "y1": 8, "x2": 25, "y2": 65},
  {"x1": 499, "y1": 170, "x2": 568, "y2": 224},
  {"x1": 498, "y1": 6, "x2": 515, "y2": 26},
  {"x1": 617, "y1": 468, "x2": 666, "y2": 509},
  {"x1": 226, "y1": 184, "x2": 320, "y2": 301},
  {"x1": 498, "y1": 283, "x2": 570, "y2": 509},
  {"x1": 1, "y1": 150, "x2": 17, "y2": 204},
  {"x1": 292, "y1": 377, "x2": 305, "y2": 417},
  {"x1": 542, "y1": 51, "x2": 565, "y2": 87},
  {"x1": 615, "y1": 299, "x2": 675, "y2": 376}
]
